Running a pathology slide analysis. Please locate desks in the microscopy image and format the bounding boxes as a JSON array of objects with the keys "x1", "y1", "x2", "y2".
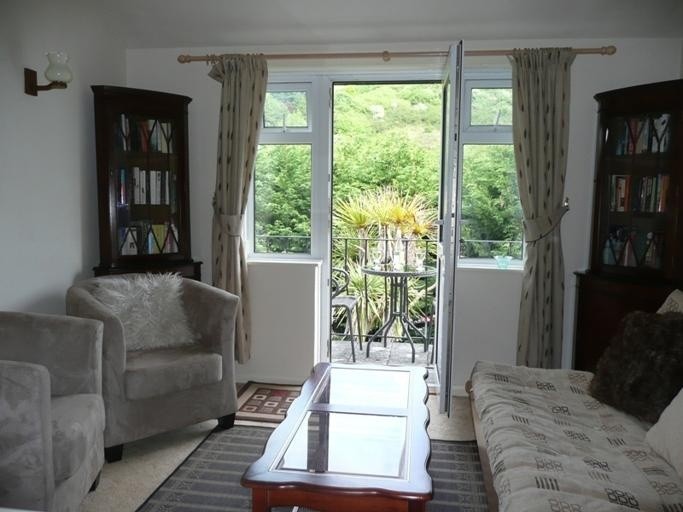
[{"x1": 360, "y1": 266, "x2": 435, "y2": 363}]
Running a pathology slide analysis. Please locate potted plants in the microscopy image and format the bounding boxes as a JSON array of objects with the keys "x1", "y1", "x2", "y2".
[{"x1": 330, "y1": 184, "x2": 438, "y2": 266}]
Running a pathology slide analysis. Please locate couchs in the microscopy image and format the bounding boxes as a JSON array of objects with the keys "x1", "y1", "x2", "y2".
[{"x1": 462, "y1": 288, "x2": 682, "y2": 512}]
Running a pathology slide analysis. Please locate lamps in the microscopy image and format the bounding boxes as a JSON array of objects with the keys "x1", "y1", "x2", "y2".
[{"x1": 22, "y1": 52, "x2": 73, "y2": 97}]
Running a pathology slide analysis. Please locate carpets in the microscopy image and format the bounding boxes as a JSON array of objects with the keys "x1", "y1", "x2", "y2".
[
  {"x1": 136, "y1": 423, "x2": 492, "y2": 512},
  {"x1": 231, "y1": 379, "x2": 302, "y2": 424}
]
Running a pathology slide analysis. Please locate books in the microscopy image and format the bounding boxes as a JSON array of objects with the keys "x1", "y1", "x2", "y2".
[
  {"x1": 107, "y1": 108, "x2": 180, "y2": 257},
  {"x1": 594, "y1": 107, "x2": 676, "y2": 271}
]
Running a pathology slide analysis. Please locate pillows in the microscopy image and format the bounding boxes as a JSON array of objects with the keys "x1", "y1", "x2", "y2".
[{"x1": 587, "y1": 307, "x2": 682, "y2": 478}]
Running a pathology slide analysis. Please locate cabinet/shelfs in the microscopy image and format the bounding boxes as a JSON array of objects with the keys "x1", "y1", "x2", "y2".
[
  {"x1": 569, "y1": 76, "x2": 682, "y2": 373},
  {"x1": 89, "y1": 83, "x2": 203, "y2": 280}
]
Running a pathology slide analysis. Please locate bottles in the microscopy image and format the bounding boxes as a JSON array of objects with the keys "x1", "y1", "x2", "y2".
[{"x1": 601, "y1": 227, "x2": 657, "y2": 267}]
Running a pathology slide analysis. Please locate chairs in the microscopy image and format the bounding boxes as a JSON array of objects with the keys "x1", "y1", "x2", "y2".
[
  {"x1": 64, "y1": 273, "x2": 240, "y2": 463},
  {"x1": 329, "y1": 267, "x2": 363, "y2": 362},
  {"x1": 0, "y1": 307, "x2": 111, "y2": 512}
]
